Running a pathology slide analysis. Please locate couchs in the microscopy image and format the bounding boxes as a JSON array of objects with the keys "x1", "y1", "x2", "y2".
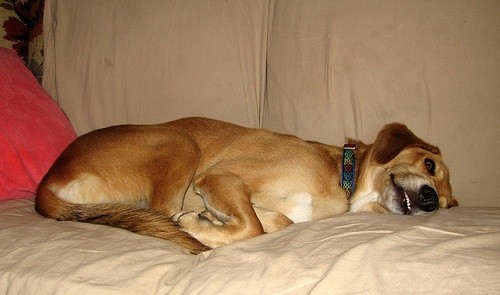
[{"x1": 0, "y1": 0, "x2": 500, "y2": 294}]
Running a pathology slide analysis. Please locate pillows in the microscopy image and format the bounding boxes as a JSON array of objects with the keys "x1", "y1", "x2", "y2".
[{"x1": 0, "y1": 47, "x2": 79, "y2": 200}]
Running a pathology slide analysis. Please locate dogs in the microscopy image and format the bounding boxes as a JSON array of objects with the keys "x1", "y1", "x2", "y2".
[{"x1": 34, "y1": 117, "x2": 460, "y2": 256}]
